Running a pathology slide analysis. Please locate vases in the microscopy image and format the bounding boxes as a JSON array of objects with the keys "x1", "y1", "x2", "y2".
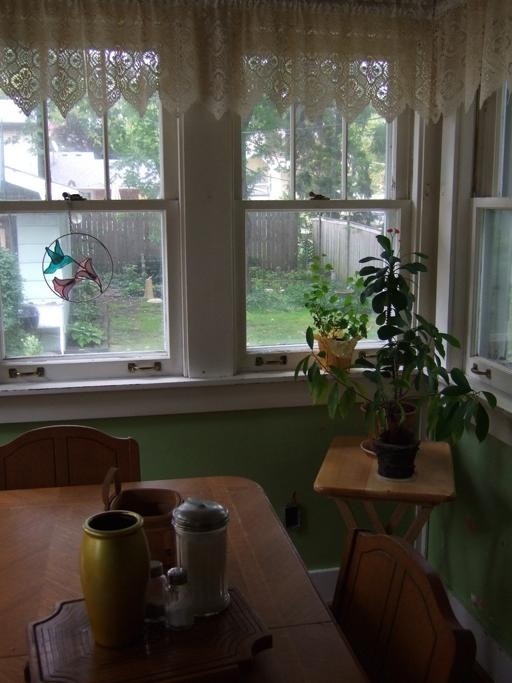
[
  {"x1": 315, "y1": 334, "x2": 356, "y2": 373},
  {"x1": 375, "y1": 432, "x2": 419, "y2": 481},
  {"x1": 361, "y1": 400, "x2": 417, "y2": 458}
]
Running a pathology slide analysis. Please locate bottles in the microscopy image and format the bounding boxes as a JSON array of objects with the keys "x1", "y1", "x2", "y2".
[
  {"x1": 82, "y1": 511, "x2": 149, "y2": 645},
  {"x1": 164, "y1": 567, "x2": 195, "y2": 632},
  {"x1": 173, "y1": 497, "x2": 232, "y2": 618},
  {"x1": 150, "y1": 560, "x2": 166, "y2": 619}
]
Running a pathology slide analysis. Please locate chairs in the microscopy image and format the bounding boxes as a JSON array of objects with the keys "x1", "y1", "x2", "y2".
[
  {"x1": 332, "y1": 529, "x2": 476, "y2": 683},
  {"x1": 0, "y1": 424, "x2": 141, "y2": 491}
]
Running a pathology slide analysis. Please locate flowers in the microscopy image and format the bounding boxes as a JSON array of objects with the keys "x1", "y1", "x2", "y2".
[
  {"x1": 305, "y1": 246, "x2": 369, "y2": 341},
  {"x1": 295, "y1": 228, "x2": 497, "y2": 439}
]
